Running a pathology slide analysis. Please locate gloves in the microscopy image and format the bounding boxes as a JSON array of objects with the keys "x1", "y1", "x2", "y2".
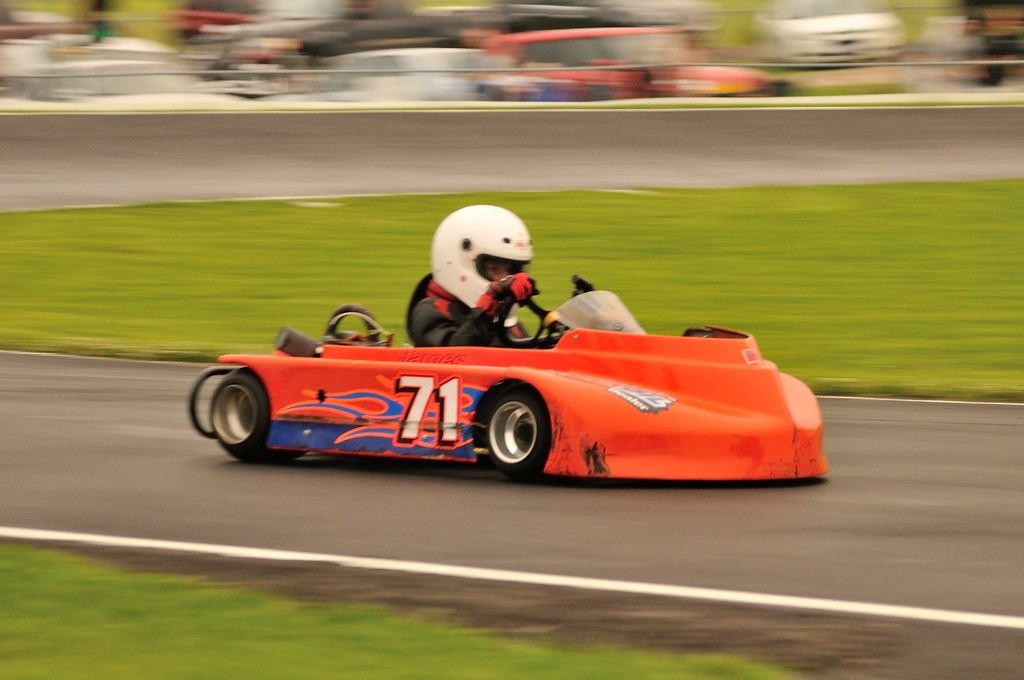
[{"x1": 476, "y1": 273, "x2": 541, "y2": 316}]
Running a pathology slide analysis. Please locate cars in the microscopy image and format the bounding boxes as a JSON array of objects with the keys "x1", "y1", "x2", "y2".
[{"x1": 743, "y1": 0, "x2": 904, "y2": 73}]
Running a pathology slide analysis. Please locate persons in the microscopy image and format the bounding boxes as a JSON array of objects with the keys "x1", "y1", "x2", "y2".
[{"x1": 410, "y1": 204, "x2": 591, "y2": 351}]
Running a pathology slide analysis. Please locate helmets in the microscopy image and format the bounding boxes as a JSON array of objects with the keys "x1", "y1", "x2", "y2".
[{"x1": 432, "y1": 204, "x2": 534, "y2": 307}]
